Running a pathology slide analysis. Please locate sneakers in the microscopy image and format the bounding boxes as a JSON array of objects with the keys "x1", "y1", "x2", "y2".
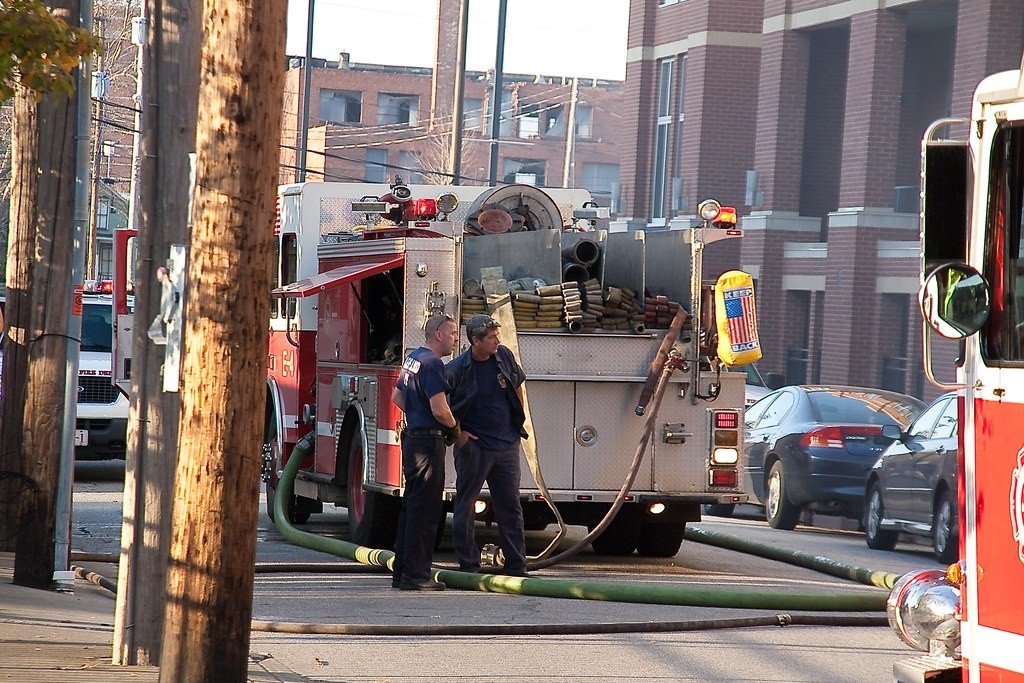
[{"x1": 392, "y1": 574, "x2": 445, "y2": 591}]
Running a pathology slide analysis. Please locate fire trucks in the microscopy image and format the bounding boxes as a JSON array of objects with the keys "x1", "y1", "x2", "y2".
[
  {"x1": 886, "y1": 48, "x2": 1024, "y2": 683},
  {"x1": 112, "y1": 175, "x2": 764, "y2": 559}
]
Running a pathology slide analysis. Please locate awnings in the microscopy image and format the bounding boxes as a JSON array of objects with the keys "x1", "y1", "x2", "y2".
[{"x1": 270, "y1": 256, "x2": 403, "y2": 332}]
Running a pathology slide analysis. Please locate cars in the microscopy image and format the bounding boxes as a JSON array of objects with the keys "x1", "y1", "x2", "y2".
[
  {"x1": 703, "y1": 385, "x2": 929, "y2": 531},
  {"x1": 863, "y1": 390, "x2": 960, "y2": 565}
]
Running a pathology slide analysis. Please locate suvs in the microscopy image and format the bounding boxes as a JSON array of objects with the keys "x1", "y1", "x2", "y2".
[{"x1": 72, "y1": 280, "x2": 136, "y2": 462}]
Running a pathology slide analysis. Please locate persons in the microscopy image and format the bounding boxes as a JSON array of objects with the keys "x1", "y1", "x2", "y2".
[
  {"x1": 392, "y1": 315, "x2": 461, "y2": 591},
  {"x1": 445, "y1": 314, "x2": 541, "y2": 581}
]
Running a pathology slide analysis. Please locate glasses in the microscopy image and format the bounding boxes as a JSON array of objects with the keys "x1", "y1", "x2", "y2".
[
  {"x1": 436, "y1": 314, "x2": 451, "y2": 330},
  {"x1": 469, "y1": 319, "x2": 500, "y2": 330}
]
